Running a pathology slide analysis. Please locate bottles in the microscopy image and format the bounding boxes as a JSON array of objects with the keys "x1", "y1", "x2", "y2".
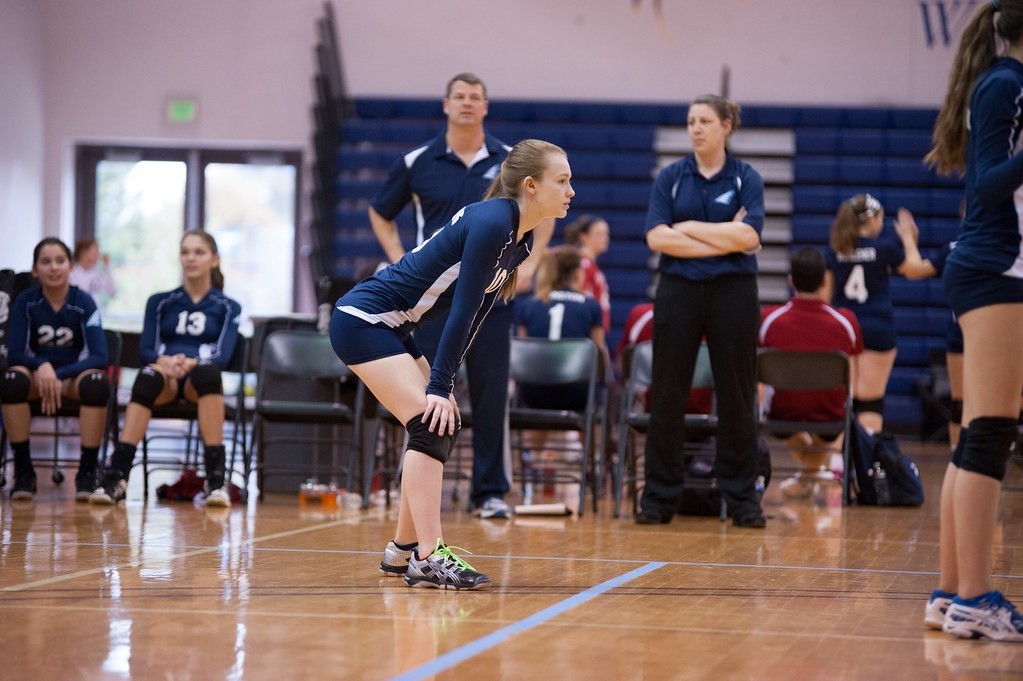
[
  {"x1": 874, "y1": 461, "x2": 890, "y2": 507},
  {"x1": 523, "y1": 428, "x2": 583, "y2": 497}
]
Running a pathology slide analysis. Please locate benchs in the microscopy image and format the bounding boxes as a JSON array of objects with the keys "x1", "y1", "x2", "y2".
[{"x1": 307, "y1": 0, "x2": 973, "y2": 446}]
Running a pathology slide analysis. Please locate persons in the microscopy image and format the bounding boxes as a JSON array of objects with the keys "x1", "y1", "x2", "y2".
[
  {"x1": 516, "y1": 245, "x2": 603, "y2": 495},
  {"x1": 892, "y1": 196, "x2": 967, "y2": 453},
  {"x1": 925, "y1": 0, "x2": 1023, "y2": 642},
  {"x1": 368, "y1": 70, "x2": 554, "y2": 520},
  {"x1": 760, "y1": 246, "x2": 864, "y2": 494},
  {"x1": 68, "y1": 235, "x2": 118, "y2": 296},
  {"x1": 328, "y1": 138, "x2": 575, "y2": 590},
  {"x1": 566, "y1": 214, "x2": 610, "y2": 462},
  {"x1": 616, "y1": 256, "x2": 715, "y2": 415},
  {"x1": 0, "y1": 237, "x2": 112, "y2": 501},
  {"x1": 89, "y1": 229, "x2": 241, "y2": 506},
  {"x1": 824, "y1": 193, "x2": 920, "y2": 478},
  {"x1": 634, "y1": 93, "x2": 772, "y2": 528}
]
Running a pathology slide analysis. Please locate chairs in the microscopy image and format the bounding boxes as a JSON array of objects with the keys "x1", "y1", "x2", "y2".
[
  {"x1": 245, "y1": 317, "x2": 854, "y2": 508},
  {"x1": 140, "y1": 332, "x2": 248, "y2": 502},
  {"x1": 0, "y1": 327, "x2": 122, "y2": 488}
]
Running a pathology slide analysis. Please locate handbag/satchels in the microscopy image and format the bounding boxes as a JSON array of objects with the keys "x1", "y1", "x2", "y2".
[{"x1": 680, "y1": 432, "x2": 772, "y2": 513}]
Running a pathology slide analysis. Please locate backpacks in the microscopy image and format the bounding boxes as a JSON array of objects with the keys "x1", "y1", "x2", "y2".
[{"x1": 849, "y1": 419, "x2": 923, "y2": 506}]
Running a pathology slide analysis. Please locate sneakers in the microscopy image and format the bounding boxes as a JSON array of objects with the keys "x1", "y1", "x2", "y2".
[
  {"x1": 379, "y1": 577, "x2": 408, "y2": 608},
  {"x1": 924, "y1": 589, "x2": 957, "y2": 627},
  {"x1": 10, "y1": 469, "x2": 37, "y2": 499},
  {"x1": 473, "y1": 498, "x2": 511, "y2": 518},
  {"x1": 205, "y1": 475, "x2": 230, "y2": 508},
  {"x1": 924, "y1": 629, "x2": 1022, "y2": 676},
  {"x1": 404, "y1": 538, "x2": 491, "y2": 590},
  {"x1": 942, "y1": 590, "x2": 1023, "y2": 642},
  {"x1": 74, "y1": 470, "x2": 95, "y2": 499},
  {"x1": 406, "y1": 590, "x2": 492, "y2": 633},
  {"x1": 88, "y1": 469, "x2": 128, "y2": 504},
  {"x1": 380, "y1": 540, "x2": 418, "y2": 577}
]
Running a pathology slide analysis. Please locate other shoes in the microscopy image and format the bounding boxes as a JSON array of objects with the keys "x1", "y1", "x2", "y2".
[
  {"x1": 637, "y1": 510, "x2": 666, "y2": 525},
  {"x1": 780, "y1": 474, "x2": 810, "y2": 497},
  {"x1": 733, "y1": 510, "x2": 765, "y2": 527}
]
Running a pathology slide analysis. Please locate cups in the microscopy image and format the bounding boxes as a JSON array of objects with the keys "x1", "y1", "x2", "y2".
[{"x1": 299, "y1": 483, "x2": 339, "y2": 516}]
